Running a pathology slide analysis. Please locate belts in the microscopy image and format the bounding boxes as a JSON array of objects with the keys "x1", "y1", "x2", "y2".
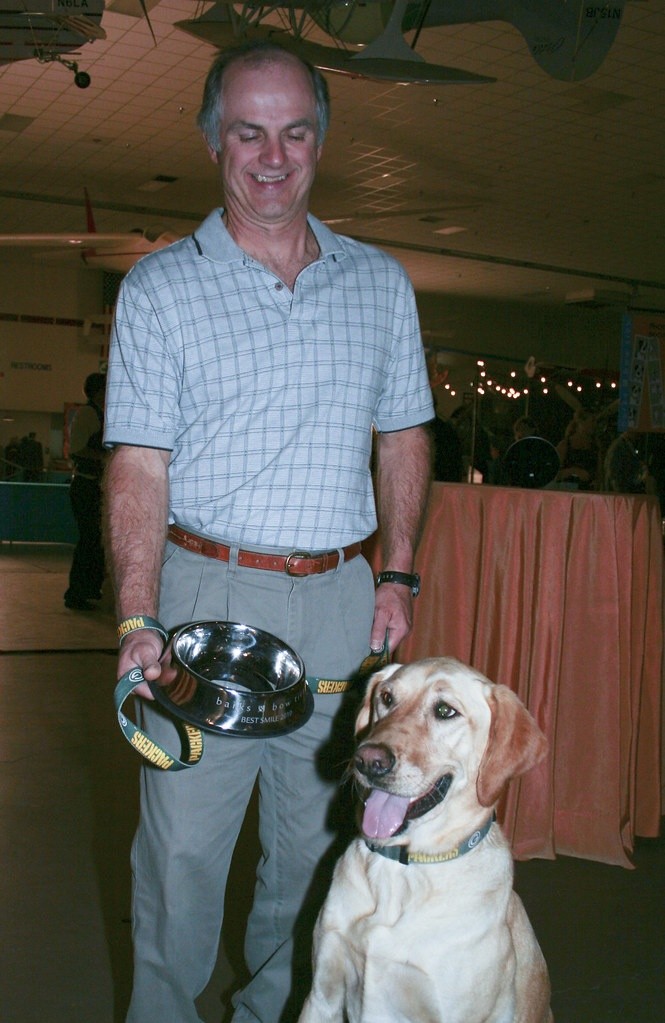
[{"x1": 167, "y1": 524, "x2": 363, "y2": 577}]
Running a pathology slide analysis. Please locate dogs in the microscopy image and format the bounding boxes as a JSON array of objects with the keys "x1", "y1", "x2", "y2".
[{"x1": 297, "y1": 655, "x2": 553, "y2": 1023}]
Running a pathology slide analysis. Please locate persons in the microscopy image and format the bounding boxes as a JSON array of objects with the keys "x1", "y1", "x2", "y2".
[
  {"x1": 433, "y1": 403, "x2": 665, "y2": 493},
  {"x1": 103, "y1": 30, "x2": 437, "y2": 1023},
  {"x1": 64, "y1": 374, "x2": 108, "y2": 612},
  {"x1": 4, "y1": 431, "x2": 44, "y2": 483}
]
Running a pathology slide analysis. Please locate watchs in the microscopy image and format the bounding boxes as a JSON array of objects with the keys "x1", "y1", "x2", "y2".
[{"x1": 374, "y1": 571, "x2": 422, "y2": 597}]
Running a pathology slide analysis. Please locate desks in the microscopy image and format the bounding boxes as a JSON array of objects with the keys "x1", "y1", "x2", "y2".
[{"x1": 366, "y1": 479, "x2": 665, "y2": 872}]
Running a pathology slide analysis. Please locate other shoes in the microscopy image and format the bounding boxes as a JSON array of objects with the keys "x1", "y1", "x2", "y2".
[
  {"x1": 87, "y1": 577, "x2": 104, "y2": 599},
  {"x1": 65, "y1": 589, "x2": 95, "y2": 611}
]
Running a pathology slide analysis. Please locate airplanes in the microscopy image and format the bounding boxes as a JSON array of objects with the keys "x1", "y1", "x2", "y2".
[{"x1": 1, "y1": 184, "x2": 492, "y2": 303}]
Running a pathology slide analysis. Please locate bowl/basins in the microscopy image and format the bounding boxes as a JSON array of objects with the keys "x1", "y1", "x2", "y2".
[{"x1": 150, "y1": 621, "x2": 314, "y2": 739}]
